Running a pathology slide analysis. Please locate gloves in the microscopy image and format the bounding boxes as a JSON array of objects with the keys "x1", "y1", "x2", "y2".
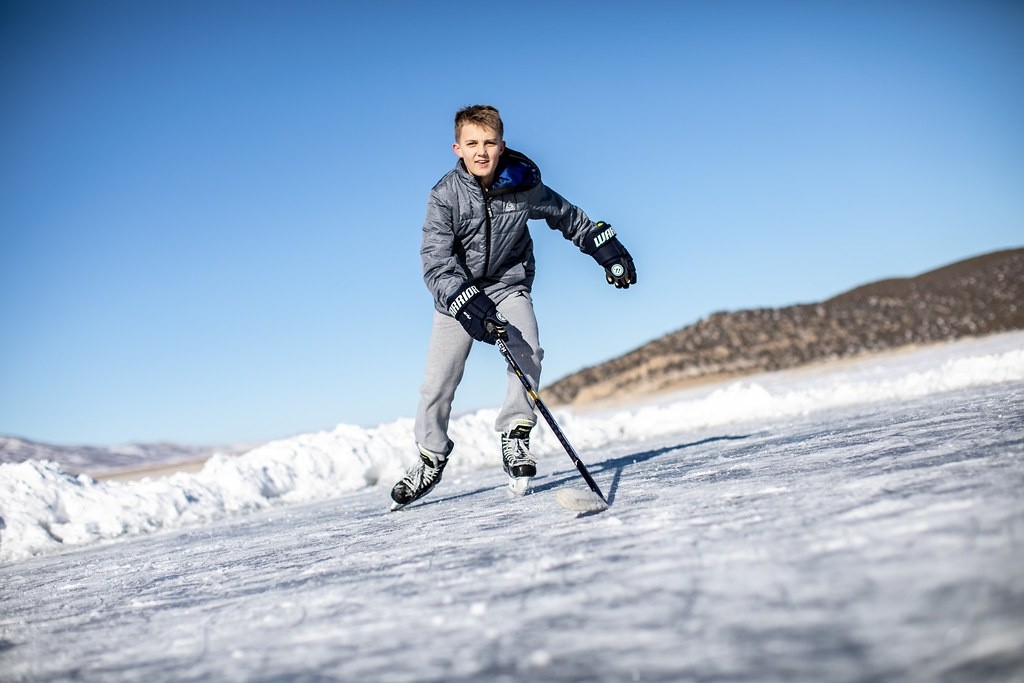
[
  {"x1": 445, "y1": 281, "x2": 509, "y2": 345},
  {"x1": 580, "y1": 221, "x2": 637, "y2": 290}
]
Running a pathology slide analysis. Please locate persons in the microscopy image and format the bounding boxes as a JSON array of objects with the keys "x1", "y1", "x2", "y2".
[{"x1": 387, "y1": 105, "x2": 638, "y2": 513}]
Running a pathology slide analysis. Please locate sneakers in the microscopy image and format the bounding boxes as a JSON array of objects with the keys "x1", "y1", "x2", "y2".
[
  {"x1": 391, "y1": 439, "x2": 454, "y2": 511},
  {"x1": 501, "y1": 419, "x2": 538, "y2": 496}
]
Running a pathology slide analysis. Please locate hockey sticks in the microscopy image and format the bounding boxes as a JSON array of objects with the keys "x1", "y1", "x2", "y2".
[{"x1": 486, "y1": 321, "x2": 609, "y2": 511}]
